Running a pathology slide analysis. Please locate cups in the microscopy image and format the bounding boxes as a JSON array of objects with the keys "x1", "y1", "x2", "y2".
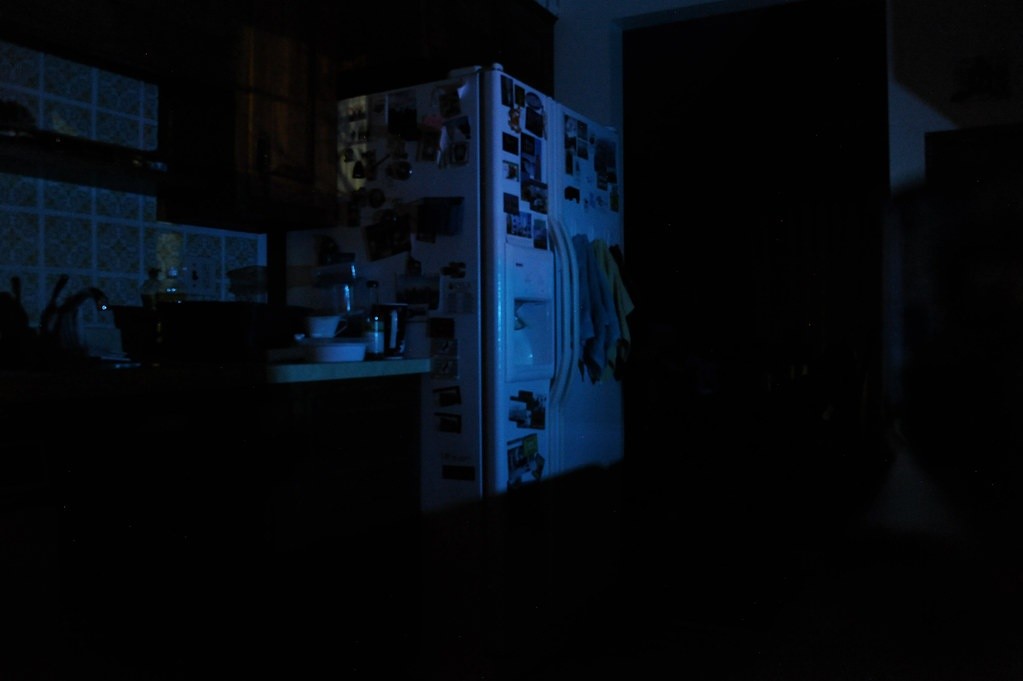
[
  {"x1": 372, "y1": 302, "x2": 408, "y2": 361},
  {"x1": 363, "y1": 315, "x2": 386, "y2": 361},
  {"x1": 308, "y1": 316, "x2": 347, "y2": 339}
]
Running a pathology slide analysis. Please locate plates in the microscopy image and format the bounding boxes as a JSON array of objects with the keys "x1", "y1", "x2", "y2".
[{"x1": 58, "y1": 288, "x2": 116, "y2": 352}]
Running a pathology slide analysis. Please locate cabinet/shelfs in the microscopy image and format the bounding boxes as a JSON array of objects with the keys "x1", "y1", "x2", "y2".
[{"x1": 221, "y1": 0, "x2": 547, "y2": 210}]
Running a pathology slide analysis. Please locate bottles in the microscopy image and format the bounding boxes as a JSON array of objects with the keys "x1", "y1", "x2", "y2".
[{"x1": 155, "y1": 267, "x2": 185, "y2": 347}]
